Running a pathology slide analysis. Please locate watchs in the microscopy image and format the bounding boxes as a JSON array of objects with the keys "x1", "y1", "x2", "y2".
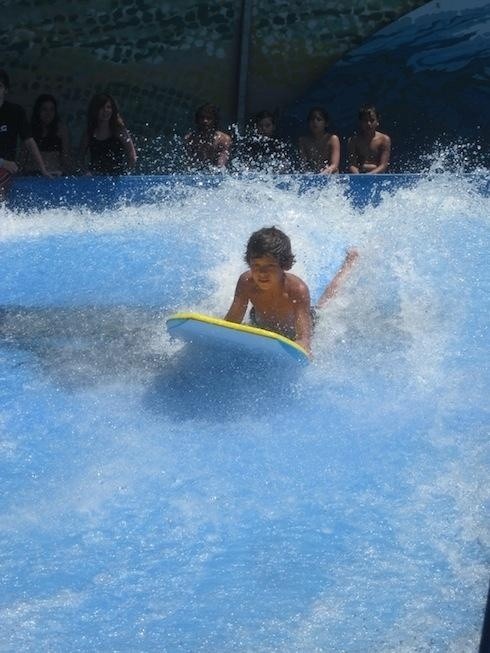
[{"x1": 62, "y1": 152, "x2": 71, "y2": 158}]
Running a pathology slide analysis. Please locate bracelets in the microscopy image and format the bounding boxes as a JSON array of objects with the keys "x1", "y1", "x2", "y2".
[
  {"x1": 38, "y1": 167, "x2": 46, "y2": 171},
  {"x1": 0, "y1": 157, "x2": 5, "y2": 168}
]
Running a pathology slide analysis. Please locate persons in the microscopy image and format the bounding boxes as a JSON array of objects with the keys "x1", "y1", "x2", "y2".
[
  {"x1": 223, "y1": 224, "x2": 360, "y2": 360},
  {"x1": 181, "y1": 100, "x2": 233, "y2": 173},
  {"x1": 292, "y1": 105, "x2": 342, "y2": 176},
  {"x1": 0, "y1": 67, "x2": 63, "y2": 180},
  {"x1": 74, "y1": 91, "x2": 137, "y2": 176},
  {"x1": 235, "y1": 109, "x2": 294, "y2": 174},
  {"x1": 17, "y1": 91, "x2": 75, "y2": 177},
  {"x1": 344, "y1": 101, "x2": 394, "y2": 175}
]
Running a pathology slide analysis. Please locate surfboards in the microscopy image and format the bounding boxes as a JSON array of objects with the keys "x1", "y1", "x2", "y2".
[{"x1": 166, "y1": 314, "x2": 311, "y2": 368}]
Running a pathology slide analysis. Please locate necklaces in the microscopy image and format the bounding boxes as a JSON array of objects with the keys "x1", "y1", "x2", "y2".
[{"x1": 97, "y1": 133, "x2": 110, "y2": 139}]
